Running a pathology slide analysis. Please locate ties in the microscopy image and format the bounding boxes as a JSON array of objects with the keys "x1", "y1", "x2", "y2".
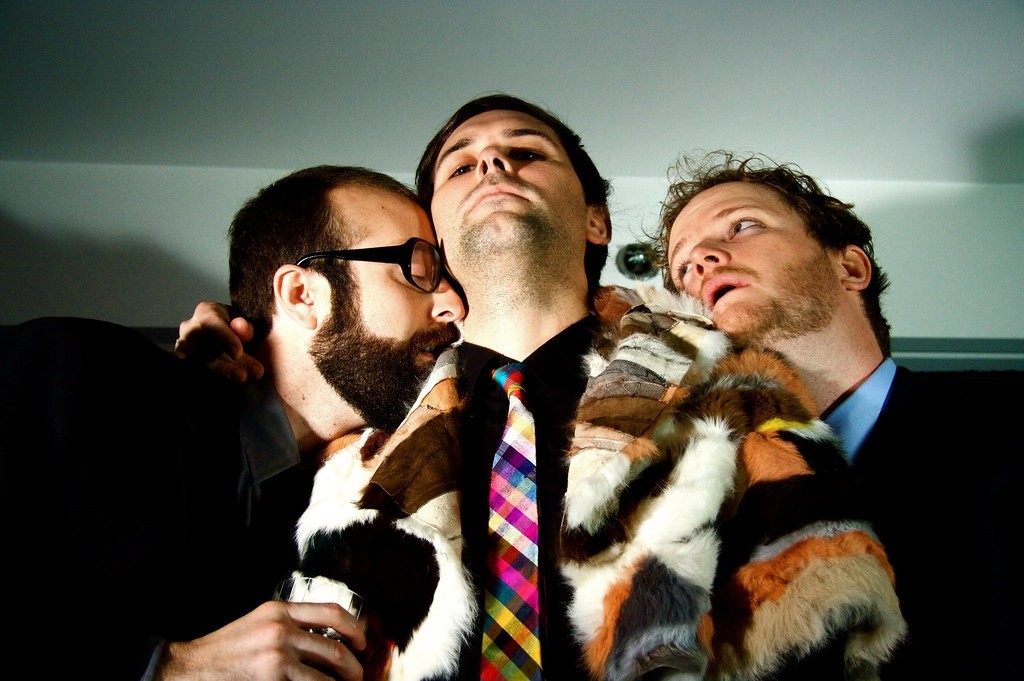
[{"x1": 480, "y1": 363, "x2": 548, "y2": 681}]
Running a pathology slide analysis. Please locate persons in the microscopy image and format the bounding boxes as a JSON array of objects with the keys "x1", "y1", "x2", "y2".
[{"x1": 0, "y1": 92, "x2": 1024, "y2": 681}]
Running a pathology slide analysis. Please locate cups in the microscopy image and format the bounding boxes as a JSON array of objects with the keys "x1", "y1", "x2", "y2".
[{"x1": 270, "y1": 577, "x2": 364, "y2": 677}]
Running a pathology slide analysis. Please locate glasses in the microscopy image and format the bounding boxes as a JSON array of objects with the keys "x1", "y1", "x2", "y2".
[{"x1": 297, "y1": 237, "x2": 449, "y2": 294}]
